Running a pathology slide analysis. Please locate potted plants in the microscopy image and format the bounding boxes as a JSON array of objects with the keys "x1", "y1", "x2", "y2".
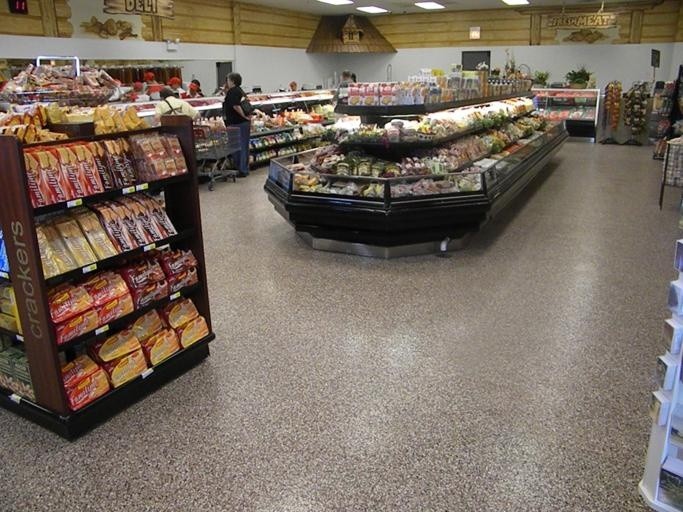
[{"x1": 563, "y1": 66, "x2": 595, "y2": 89}]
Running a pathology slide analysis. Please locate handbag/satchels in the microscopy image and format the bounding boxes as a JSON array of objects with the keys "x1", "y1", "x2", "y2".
[{"x1": 234, "y1": 87, "x2": 254, "y2": 117}]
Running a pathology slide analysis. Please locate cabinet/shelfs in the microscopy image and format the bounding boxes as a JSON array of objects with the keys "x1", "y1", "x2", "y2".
[
  {"x1": 264, "y1": 94, "x2": 572, "y2": 257},
  {"x1": 0, "y1": 113, "x2": 216, "y2": 441},
  {"x1": 532, "y1": 87, "x2": 609, "y2": 142},
  {"x1": 184, "y1": 90, "x2": 345, "y2": 183}
]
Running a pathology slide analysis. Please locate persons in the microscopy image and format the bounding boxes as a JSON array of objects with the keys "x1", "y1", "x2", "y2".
[
  {"x1": 290, "y1": 81, "x2": 297, "y2": 92},
  {"x1": 340, "y1": 70, "x2": 355, "y2": 87},
  {"x1": 134, "y1": 73, "x2": 204, "y2": 101},
  {"x1": 223, "y1": 72, "x2": 255, "y2": 174},
  {"x1": 351, "y1": 73, "x2": 357, "y2": 83}
]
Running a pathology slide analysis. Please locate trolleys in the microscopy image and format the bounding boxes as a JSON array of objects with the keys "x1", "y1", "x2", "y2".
[{"x1": 192, "y1": 125, "x2": 242, "y2": 191}]
